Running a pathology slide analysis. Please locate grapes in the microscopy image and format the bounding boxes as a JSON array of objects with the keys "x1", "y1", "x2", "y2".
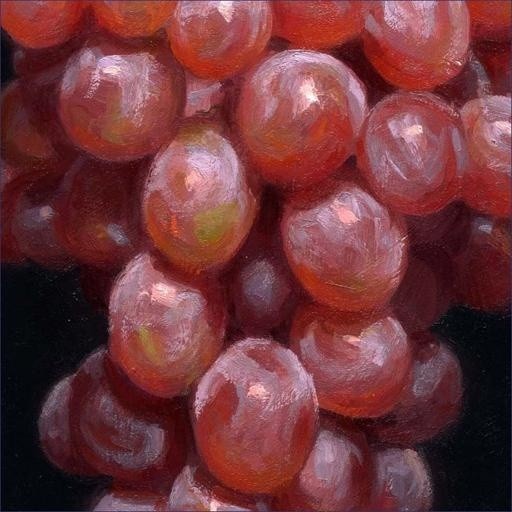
[{"x1": 0, "y1": 0, "x2": 511, "y2": 512}]
